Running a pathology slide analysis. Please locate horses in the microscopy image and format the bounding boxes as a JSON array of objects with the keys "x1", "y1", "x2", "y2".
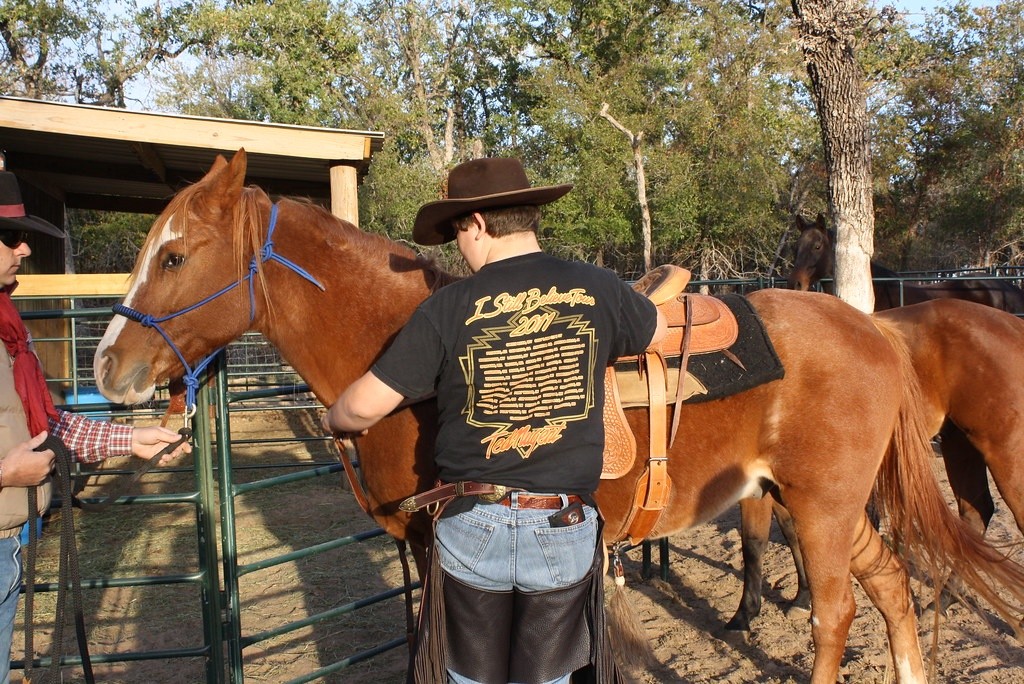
[{"x1": 92, "y1": 146, "x2": 1024, "y2": 684}]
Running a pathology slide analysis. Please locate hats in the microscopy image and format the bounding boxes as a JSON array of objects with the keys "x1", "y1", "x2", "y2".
[
  {"x1": 413, "y1": 157, "x2": 575, "y2": 245},
  {"x1": 1, "y1": 173, "x2": 68, "y2": 240}
]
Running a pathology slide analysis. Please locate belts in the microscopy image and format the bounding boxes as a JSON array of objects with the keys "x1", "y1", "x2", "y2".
[{"x1": 397, "y1": 482, "x2": 588, "y2": 513}]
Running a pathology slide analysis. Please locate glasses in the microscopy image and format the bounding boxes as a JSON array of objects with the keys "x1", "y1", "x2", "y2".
[{"x1": 1, "y1": 228, "x2": 33, "y2": 250}]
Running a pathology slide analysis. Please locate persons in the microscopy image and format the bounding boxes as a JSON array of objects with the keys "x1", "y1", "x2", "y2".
[
  {"x1": 0, "y1": 183, "x2": 198, "y2": 677},
  {"x1": 297, "y1": 155, "x2": 667, "y2": 683}
]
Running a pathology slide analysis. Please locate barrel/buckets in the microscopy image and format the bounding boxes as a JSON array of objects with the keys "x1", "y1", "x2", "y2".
[
  {"x1": 19, "y1": 517, "x2": 42, "y2": 545},
  {"x1": 62, "y1": 386, "x2": 113, "y2": 423}
]
